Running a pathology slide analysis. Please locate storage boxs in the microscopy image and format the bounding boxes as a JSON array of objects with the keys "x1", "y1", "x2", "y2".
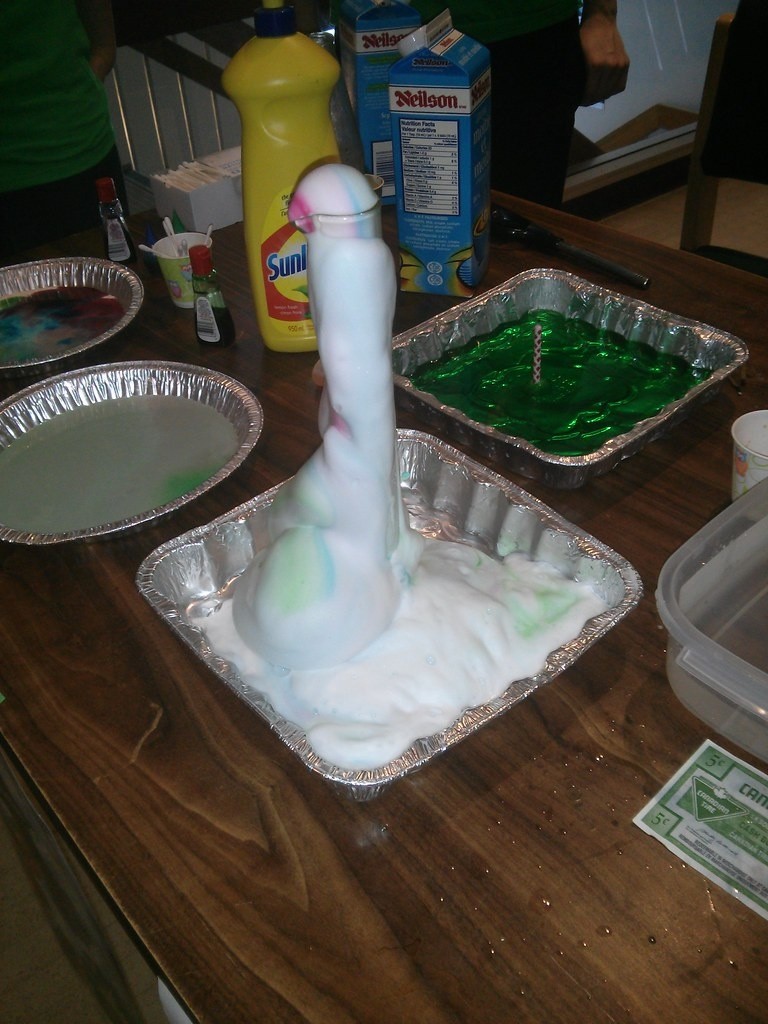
[
  {"x1": 656, "y1": 475, "x2": 766, "y2": 770},
  {"x1": 148, "y1": 142, "x2": 244, "y2": 233}
]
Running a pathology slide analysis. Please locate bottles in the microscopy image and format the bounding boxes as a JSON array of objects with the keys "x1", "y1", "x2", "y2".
[
  {"x1": 95, "y1": 176, "x2": 136, "y2": 265},
  {"x1": 308, "y1": 32, "x2": 365, "y2": 174},
  {"x1": 187, "y1": 245, "x2": 233, "y2": 349}
]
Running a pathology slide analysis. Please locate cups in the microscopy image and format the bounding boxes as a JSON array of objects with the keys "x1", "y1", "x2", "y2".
[
  {"x1": 155, "y1": 233, "x2": 212, "y2": 307},
  {"x1": 732, "y1": 410, "x2": 768, "y2": 521},
  {"x1": 365, "y1": 172, "x2": 384, "y2": 201}
]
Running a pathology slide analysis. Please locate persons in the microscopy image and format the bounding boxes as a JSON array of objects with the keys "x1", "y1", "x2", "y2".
[
  {"x1": 420, "y1": 0, "x2": 630, "y2": 208},
  {"x1": 0, "y1": 0, "x2": 129, "y2": 259}
]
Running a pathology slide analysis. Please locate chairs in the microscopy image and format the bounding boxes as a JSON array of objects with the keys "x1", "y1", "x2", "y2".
[{"x1": 680, "y1": 0, "x2": 767, "y2": 275}]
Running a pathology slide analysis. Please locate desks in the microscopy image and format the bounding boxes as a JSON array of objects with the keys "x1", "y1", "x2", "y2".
[{"x1": 0, "y1": 190, "x2": 767, "y2": 1022}]
